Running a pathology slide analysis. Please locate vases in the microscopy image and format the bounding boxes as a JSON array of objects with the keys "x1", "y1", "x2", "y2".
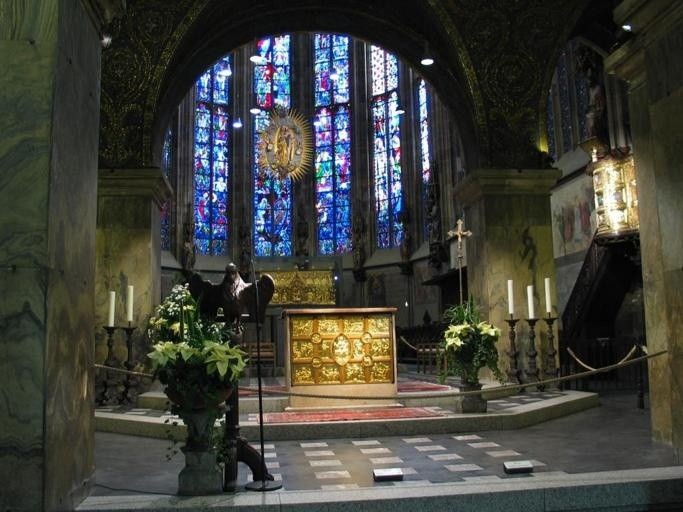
[{"x1": 452, "y1": 377, "x2": 488, "y2": 413}]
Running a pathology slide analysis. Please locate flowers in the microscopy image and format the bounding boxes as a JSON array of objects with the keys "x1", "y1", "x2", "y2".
[
  {"x1": 433, "y1": 288, "x2": 521, "y2": 395},
  {"x1": 143, "y1": 278, "x2": 227, "y2": 347}
]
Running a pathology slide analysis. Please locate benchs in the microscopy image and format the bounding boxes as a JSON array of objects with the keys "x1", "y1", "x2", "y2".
[{"x1": 414, "y1": 342, "x2": 451, "y2": 377}]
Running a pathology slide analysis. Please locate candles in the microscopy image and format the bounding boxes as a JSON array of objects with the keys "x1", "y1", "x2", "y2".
[
  {"x1": 526, "y1": 285, "x2": 535, "y2": 320},
  {"x1": 105, "y1": 290, "x2": 116, "y2": 328},
  {"x1": 544, "y1": 276, "x2": 553, "y2": 315},
  {"x1": 507, "y1": 279, "x2": 514, "y2": 315},
  {"x1": 124, "y1": 284, "x2": 134, "y2": 322}
]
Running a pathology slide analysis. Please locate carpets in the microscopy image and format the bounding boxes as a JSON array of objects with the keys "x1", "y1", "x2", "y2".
[
  {"x1": 231, "y1": 378, "x2": 453, "y2": 397},
  {"x1": 245, "y1": 401, "x2": 445, "y2": 423}
]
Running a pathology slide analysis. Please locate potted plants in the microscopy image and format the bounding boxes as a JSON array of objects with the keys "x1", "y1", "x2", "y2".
[{"x1": 140, "y1": 334, "x2": 261, "y2": 468}]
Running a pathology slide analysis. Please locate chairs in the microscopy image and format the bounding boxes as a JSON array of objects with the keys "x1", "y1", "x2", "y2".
[
  {"x1": 247, "y1": 342, "x2": 276, "y2": 382},
  {"x1": 232, "y1": 339, "x2": 251, "y2": 384}
]
